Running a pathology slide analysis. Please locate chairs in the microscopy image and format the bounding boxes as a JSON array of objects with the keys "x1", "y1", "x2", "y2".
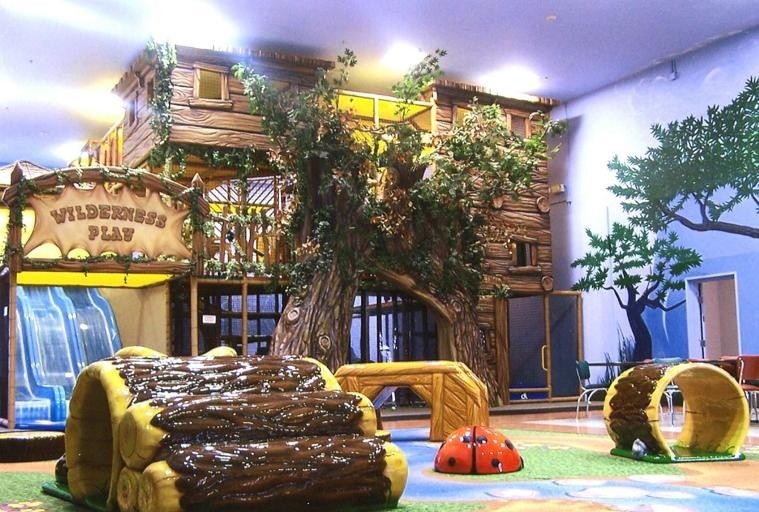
[{"x1": 575, "y1": 354, "x2": 759, "y2": 426}]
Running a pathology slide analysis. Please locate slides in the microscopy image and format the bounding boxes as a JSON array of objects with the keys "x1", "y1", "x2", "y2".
[{"x1": 15, "y1": 285, "x2": 122, "y2": 431}]
